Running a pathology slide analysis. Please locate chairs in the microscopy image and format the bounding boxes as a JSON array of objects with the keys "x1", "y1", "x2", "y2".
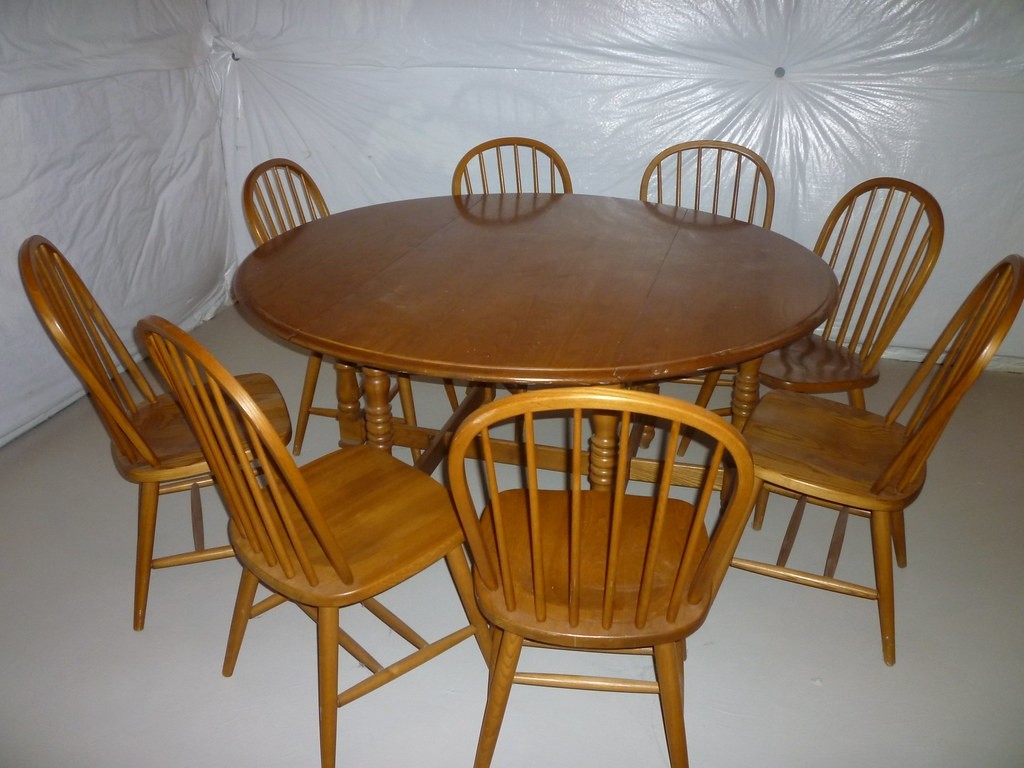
[
  {"x1": 451, "y1": 135, "x2": 574, "y2": 404},
  {"x1": 243, "y1": 158, "x2": 458, "y2": 463},
  {"x1": 447, "y1": 386, "x2": 756, "y2": 768},
  {"x1": 639, "y1": 140, "x2": 776, "y2": 389},
  {"x1": 456, "y1": 193, "x2": 565, "y2": 228},
  {"x1": 677, "y1": 177, "x2": 945, "y2": 457},
  {"x1": 720, "y1": 254, "x2": 1024, "y2": 666},
  {"x1": 17, "y1": 234, "x2": 294, "y2": 634},
  {"x1": 133, "y1": 315, "x2": 496, "y2": 768}
]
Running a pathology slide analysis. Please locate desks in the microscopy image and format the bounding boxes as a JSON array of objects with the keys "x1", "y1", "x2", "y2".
[{"x1": 230, "y1": 192, "x2": 842, "y2": 492}]
